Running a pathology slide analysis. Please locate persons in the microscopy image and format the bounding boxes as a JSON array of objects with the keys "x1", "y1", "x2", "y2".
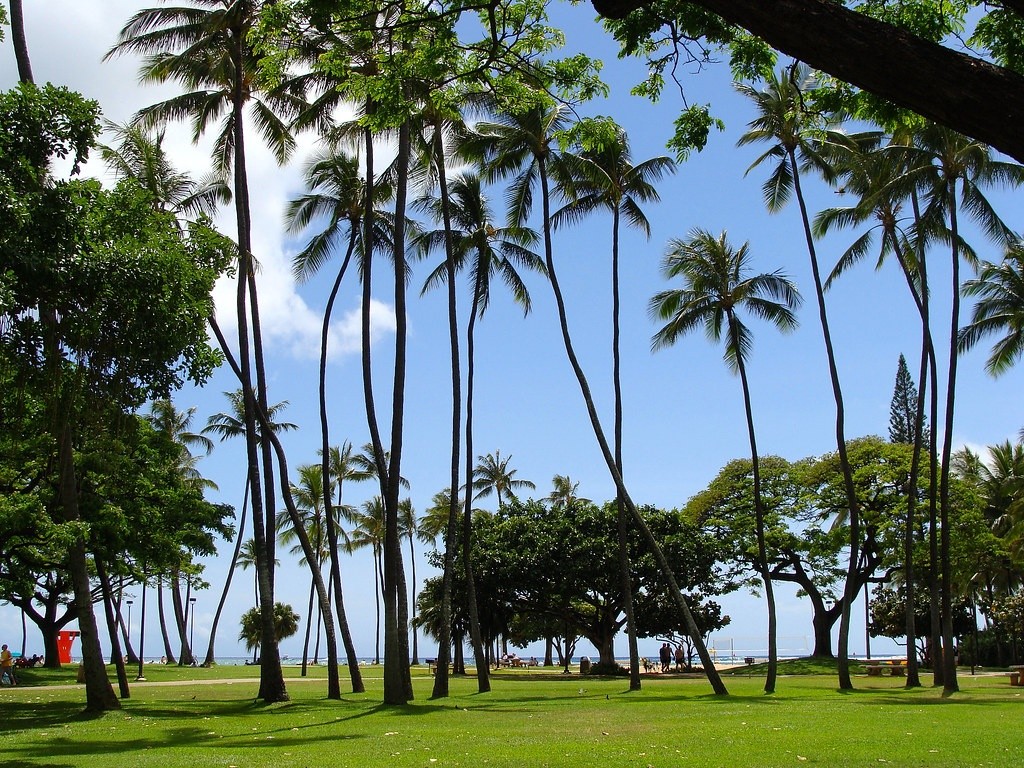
[
  {"x1": 643, "y1": 659, "x2": 659, "y2": 672},
  {"x1": 660, "y1": 643, "x2": 674, "y2": 672},
  {"x1": 0, "y1": 645, "x2": 17, "y2": 687},
  {"x1": 361, "y1": 660, "x2": 366, "y2": 666},
  {"x1": 40, "y1": 655, "x2": 44, "y2": 663},
  {"x1": 371, "y1": 660, "x2": 376, "y2": 665},
  {"x1": 124, "y1": 655, "x2": 129, "y2": 663},
  {"x1": 20, "y1": 656, "x2": 27, "y2": 668},
  {"x1": 433, "y1": 658, "x2": 438, "y2": 668},
  {"x1": 502, "y1": 653, "x2": 508, "y2": 660},
  {"x1": 530, "y1": 656, "x2": 538, "y2": 666},
  {"x1": 674, "y1": 646, "x2": 685, "y2": 672},
  {"x1": 160, "y1": 656, "x2": 166, "y2": 665}
]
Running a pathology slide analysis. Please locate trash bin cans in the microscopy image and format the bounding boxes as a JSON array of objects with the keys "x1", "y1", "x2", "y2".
[{"x1": 580, "y1": 656, "x2": 591, "y2": 673}]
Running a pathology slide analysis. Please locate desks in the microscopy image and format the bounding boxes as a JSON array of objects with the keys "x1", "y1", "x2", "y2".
[
  {"x1": 858, "y1": 658, "x2": 908, "y2": 678},
  {"x1": 1009, "y1": 665, "x2": 1024, "y2": 685}
]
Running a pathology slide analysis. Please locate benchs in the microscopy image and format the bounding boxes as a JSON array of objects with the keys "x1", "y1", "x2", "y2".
[
  {"x1": 1005, "y1": 673, "x2": 1020, "y2": 686},
  {"x1": 859, "y1": 664, "x2": 906, "y2": 670},
  {"x1": 432, "y1": 667, "x2": 466, "y2": 675}
]
